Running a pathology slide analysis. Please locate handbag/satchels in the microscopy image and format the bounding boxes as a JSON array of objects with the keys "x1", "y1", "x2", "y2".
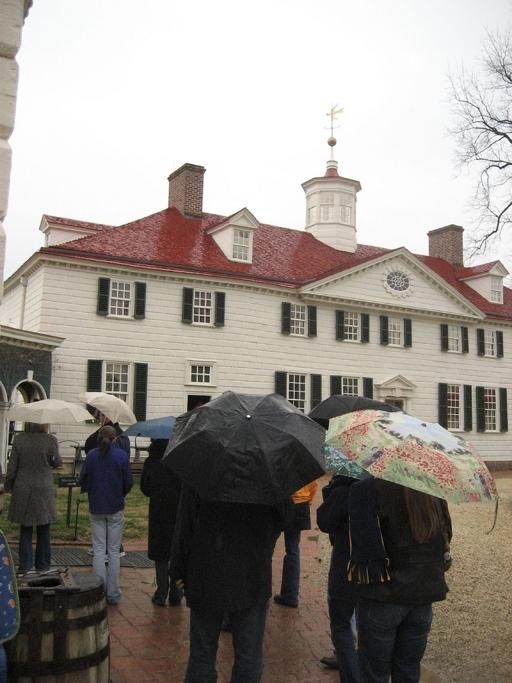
[{"x1": 336, "y1": 557, "x2": 393, "y2": 599}]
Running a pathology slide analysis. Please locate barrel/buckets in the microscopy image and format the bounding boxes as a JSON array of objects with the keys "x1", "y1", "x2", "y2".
[{"x1": 5, "y1": 569, "x2": 110, "y2": 683}]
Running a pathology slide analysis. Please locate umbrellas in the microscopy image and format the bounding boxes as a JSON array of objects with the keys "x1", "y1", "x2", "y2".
[
  {"x1": 160, "y1": 390, "x2": 327, "y2": 506},
  {"x1": 120, "y1": 415, "x2": 176, "y2": 440},
  {"x1": 2, "y1": 399, "x2": 97, "y2": 425},
  {"x1": 322, "y1": 409, "x2": 500, "y2": 503},
  {"x1": 73, "y1": 392, "x2": 137, "y2": 433},
  {"x1": 307, "y1": 394, "x2": 404, "y2": 431}
]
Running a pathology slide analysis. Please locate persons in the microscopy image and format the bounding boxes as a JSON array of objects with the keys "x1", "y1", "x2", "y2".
[
  {"x1": 3, "y1": 422, "x2": 64, "y2": 572},
  {"x1": 168, "y1": 480, "x2": 296, "y2": 683},
  {"x1": 347, "y1": 477, "x2": 453, "y2": 683},
  {"x1": 141, "y1": 437, "x2": 184, "y2": 607},
  {"x1": 78, "y1": 426, "x2": 133, "y2": 606},
  {"x1": 315, "y1": 474, "x2": 362, "y2": 683},
  {"x1": 273, "y1": 479, "x2": 318, "y2": 608},
  {"x1": 85, "y1": 410, "x2": 130, "y2": 559}
]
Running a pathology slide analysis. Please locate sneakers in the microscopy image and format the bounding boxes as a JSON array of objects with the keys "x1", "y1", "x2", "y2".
[{"x1": 274, "y1": 594, "x2": 298, "y2": 607}]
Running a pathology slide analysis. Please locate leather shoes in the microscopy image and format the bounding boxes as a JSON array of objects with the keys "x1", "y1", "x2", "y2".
[{"x1": 320, "y1": 656, "x2": 338, "y2": 666}]
